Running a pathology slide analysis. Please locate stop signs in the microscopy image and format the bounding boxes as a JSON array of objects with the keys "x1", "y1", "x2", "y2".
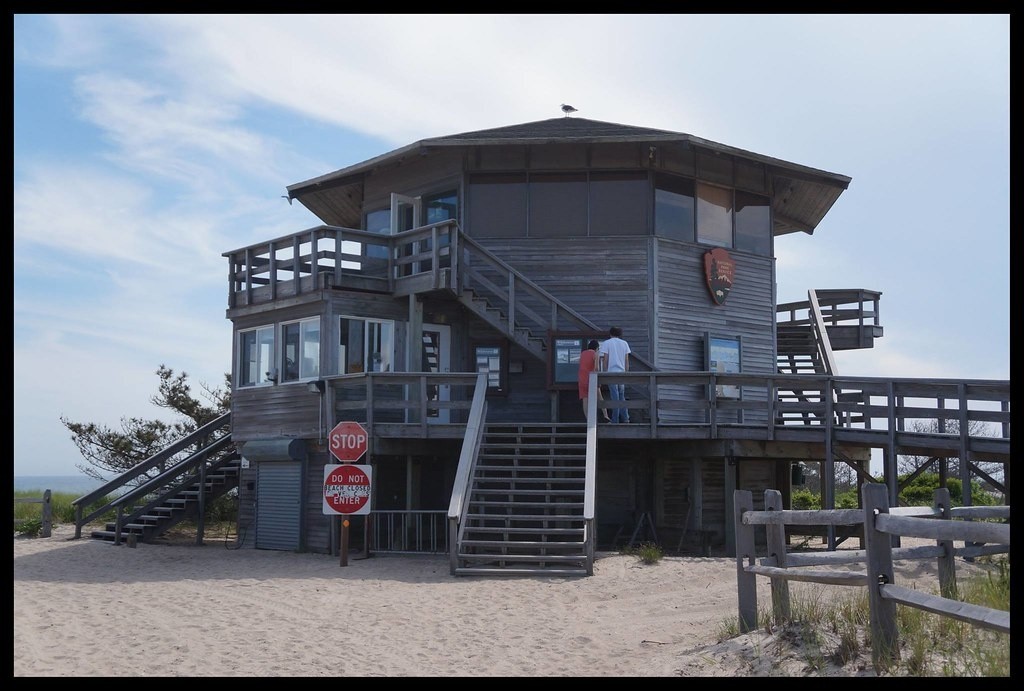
[
  {"x1": 322, "y1": 464, "x2": 372, "y2": 515},
  {"x1": 329, "y1": 421, "x2": 368, "y2": 462}
]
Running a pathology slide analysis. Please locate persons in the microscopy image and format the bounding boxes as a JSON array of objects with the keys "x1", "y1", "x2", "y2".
[
  {"x1": 579, "y1": 340, "x2": 612, "y2": 422},
  {"x1": 601, "y1": 327, "x2": 631, "y2": 423}
]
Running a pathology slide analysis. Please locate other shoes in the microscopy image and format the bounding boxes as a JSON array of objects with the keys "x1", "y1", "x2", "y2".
[
  {"x1": 603, "y1": 416, "x2": 611, "y2": 422},
  {"x1": 619, "y1": 419, "x2": 631, "y2": 423}
]
